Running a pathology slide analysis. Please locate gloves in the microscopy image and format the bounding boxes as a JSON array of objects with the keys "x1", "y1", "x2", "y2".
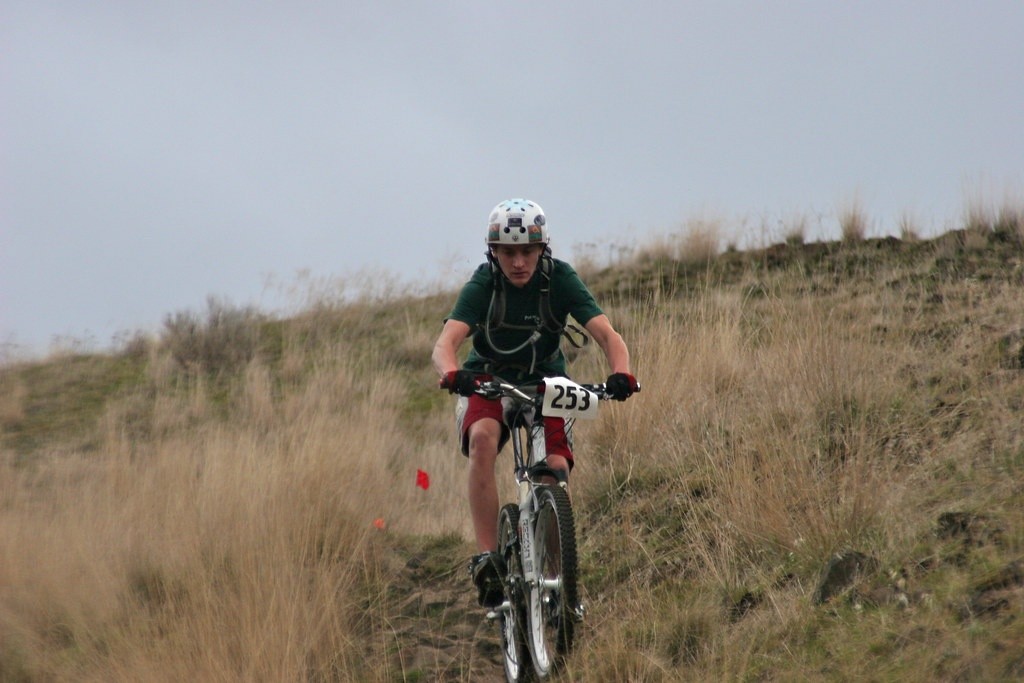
[
  {"x1": 605, "y1": 373, "x2": 638, "y2": 401},
  {"x1": 444, "y1": 371, "x2": 473, "y2": 398}
]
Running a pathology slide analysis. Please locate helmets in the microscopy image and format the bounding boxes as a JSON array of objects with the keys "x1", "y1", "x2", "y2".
[{"x1": 487, "y1": 198, "x2": 548, "y2": 244}]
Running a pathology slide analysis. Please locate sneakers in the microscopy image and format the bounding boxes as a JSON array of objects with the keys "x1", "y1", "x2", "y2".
[
  {"x1": 548, "y1": 592, "x2": 583, "y2": 625},
  {"x1": 472, "y1": 553, "x2": 509, "y2": 607}
]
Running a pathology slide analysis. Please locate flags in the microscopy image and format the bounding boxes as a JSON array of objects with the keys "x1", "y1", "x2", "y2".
[{"x1": 416, "y1": 468, "x2": 429, "y2": 490}]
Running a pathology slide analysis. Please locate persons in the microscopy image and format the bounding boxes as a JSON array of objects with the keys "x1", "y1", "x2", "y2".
[{"x1": 431, "y1": 198, "x2": 636, "y2": 625}]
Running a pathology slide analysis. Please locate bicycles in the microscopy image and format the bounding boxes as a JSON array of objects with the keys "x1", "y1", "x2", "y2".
[{"x1": 435, "y1": 376, "x2": 642, "y2": 682}]
